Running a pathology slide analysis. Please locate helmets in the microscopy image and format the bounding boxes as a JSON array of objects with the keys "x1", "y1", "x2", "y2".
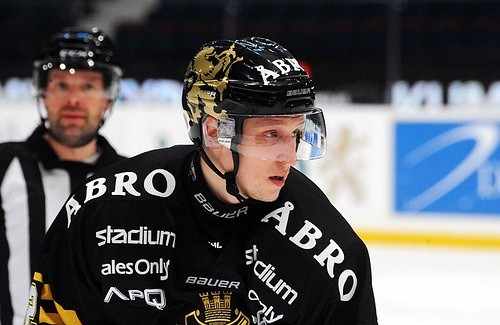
[
  {"x1": 28, "y1": 26, "x2": 123, "y2": 90},
  {"x1": 182, "y1": 36, "x2": 315, "y2": 144}
]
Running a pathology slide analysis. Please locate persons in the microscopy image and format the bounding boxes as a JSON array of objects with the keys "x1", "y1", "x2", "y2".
[
  {"x1": 23, "y1": 36, "x2": 379, "y2": 325},
  {"x1": 0, "y1": 27, "x2": 132, "y2": 325}
]
